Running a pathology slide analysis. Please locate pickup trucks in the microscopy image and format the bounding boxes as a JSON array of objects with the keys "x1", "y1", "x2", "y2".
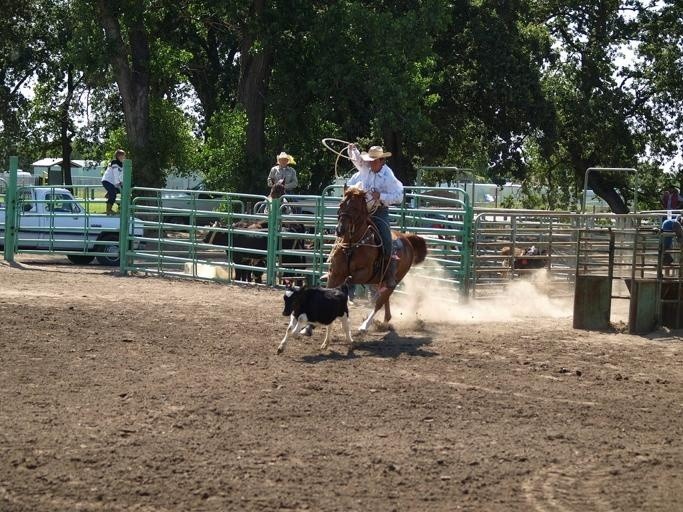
[
  {"x1": 263, "y1": 178, "x2": 443, "y2": 244},
  {"x1": 0, "y1": 184, "x2": 146, "y2": 267}
]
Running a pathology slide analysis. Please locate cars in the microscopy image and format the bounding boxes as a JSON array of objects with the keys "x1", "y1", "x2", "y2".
[{"x1": 162, "y1": 178, "x2": 227, "y2": 232}]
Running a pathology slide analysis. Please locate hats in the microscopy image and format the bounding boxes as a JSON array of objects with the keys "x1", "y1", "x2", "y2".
[
  {"x1": 276, "y1": 152, "x2": 289, "y2": 165},
  {"x1": 359, "y1": 146, "x2": 392, "y2": 161}
]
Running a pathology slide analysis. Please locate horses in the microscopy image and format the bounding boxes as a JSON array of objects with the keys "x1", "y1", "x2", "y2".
[
  {"x1": 298, "y1": 182, "x2": 428, "y2": 337},
  {"x1": 264, "y1": 176, "x2": 304, "y2": 286}
]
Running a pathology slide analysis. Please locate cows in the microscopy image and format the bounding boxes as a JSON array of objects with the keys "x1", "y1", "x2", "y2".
[
  {"x1": 203, "y1": 221, "x2": 307, "y2": 284},
  {"x1": 276, "y1": 275, "x2": 353, "y2": 355}
]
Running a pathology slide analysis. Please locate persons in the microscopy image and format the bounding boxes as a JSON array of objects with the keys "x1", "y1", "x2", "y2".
[
  {"x1": 100, "y1": 149, "x2": 126, "y2": 217},
  {"x1": 661, "y1": 186, "x2": 682, "y2": 222},
  {"x1": 319, "y1": 144, "x2": 403, "y2": 290},
  {"x1": 656, "y1": 218, "x2": 682, "y2": 276},
  {"x1": 500, "y1": 245, "x2": 515, "y2": 293},
  {"x1": 267, "y1": 152, "x2": 304, "y2": 229}
]
